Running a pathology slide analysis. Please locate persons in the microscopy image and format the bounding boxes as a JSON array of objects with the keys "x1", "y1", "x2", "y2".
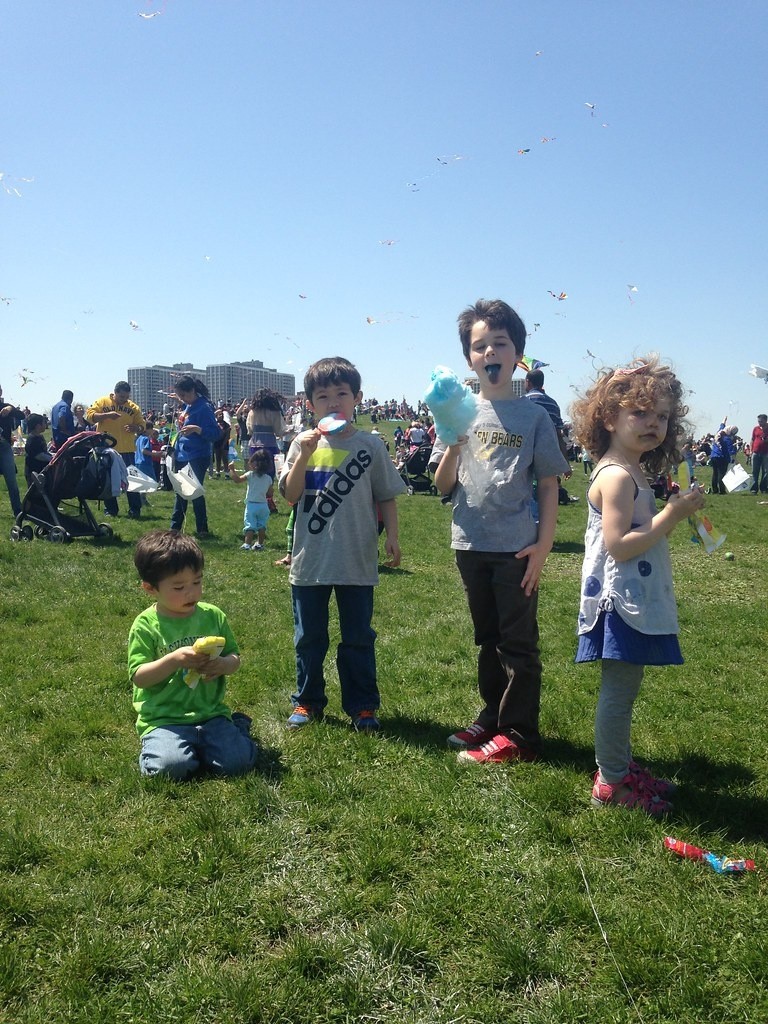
[
  {"x1": 277, "y1": 356, "x2": 408, "y2": 733},
  {"x1": 14, "y1": 381, "x2": 452, "y2": 570},
  {"x1": 0, "y1": 385, "x2": 26, "y2": 524},
  {"x1": 567, "y1": 350, "x2": 707, "y2": 815},
  {"x1": 170, "y1": 375, "x2": 225, "y2": 535},
  {"x1": 553, "y1": 413, "x2": 768, "y2": 505},
  {"x1": 427, "y1": 298, "x2": 570, "y2": 764},
  {"x1": 128, "y1": 530, "x2": 259, "y2": 783},
  {"x1": 522, "y1": 369, "x2": 573, "y2": 481}
]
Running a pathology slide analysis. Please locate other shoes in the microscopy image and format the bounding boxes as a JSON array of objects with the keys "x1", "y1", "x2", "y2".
[
  {"x1": 217, "y1": 474, "x2": 220, "y2": 479},
  {"x1": 209, "y1": 475, "x2": 213, "y2": 478},
  {"x1": 253, "y1": 544, "x2": 263, "y2": 550},
  {"x1": 241, "y1": 544, "x2": 250, "y2": 551},
  {"x1": 232, "y1": 712, "x2": 250, "y2": 727},
  {"x1": 225, "y1": 475, "x2": 229, "y2": 480}
]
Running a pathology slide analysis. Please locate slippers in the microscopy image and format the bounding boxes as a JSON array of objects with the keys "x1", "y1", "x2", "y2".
[{"x1": 275, "y1": 559, "x2": 291, "y2": 566}]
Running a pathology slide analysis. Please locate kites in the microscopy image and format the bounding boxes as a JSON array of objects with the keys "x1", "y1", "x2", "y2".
[
  {"x1": 628, "y1": 285, "x2": 638, "y2": 305},
  {"x1": 535, "y1": 50, "x2": 542, "y2": 57},
  {"x1": 686, "y1": 390, "x2": 696, "y2": 397},
  {"x1": 527, "y1": 290, "x2": 568, "y2": 340},
  {"x1": 204, "y1": 255, "x2": 379, "y2": 325},
  {"x1": 379, "y1": 102, "x2": 611, "y2": 246},
  {"x1": 517, "y1": 354, "x2": 550, "y2": 372},
  {"x1": 748, "y1": 364, "x2": 768, "y2": 385},
  {"x1": 583, "y1": 349, "x2": 596, "y2": 360}
]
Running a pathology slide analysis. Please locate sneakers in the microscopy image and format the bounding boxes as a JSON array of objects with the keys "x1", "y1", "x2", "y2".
[
  {"x1": 351, "y1": 711, "x2": 379, "y2": 735},
  {"x1": 287, "y1": 706, "x2": 323, "y2": 728},
  {"x1": 456, "y1": 731, "x2": 536, "y2": 765},
  {"x1": 447, "y1": 719, "x2": 495, "y2": 748}
]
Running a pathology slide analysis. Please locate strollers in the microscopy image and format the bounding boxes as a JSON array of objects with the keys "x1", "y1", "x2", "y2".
[
  {"x1": 10, "y1": 431, "x2": 118, "y2": 542},
  {"x1": 398, "y1": 443, "x2": 438, "y2": 496}
]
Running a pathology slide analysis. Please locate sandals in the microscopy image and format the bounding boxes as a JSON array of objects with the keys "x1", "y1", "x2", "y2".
[
  {"x1": 591, "y1": 761, "x2": 674, "y2": 817},
  {"x1": 595, "y1": 762, "x2": 673, "y2": 797}
]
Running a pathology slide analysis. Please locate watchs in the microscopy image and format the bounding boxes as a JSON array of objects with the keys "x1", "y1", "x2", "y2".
[{"x1": 138, "y1": 424, "x2": 141, "y2": 432}]
